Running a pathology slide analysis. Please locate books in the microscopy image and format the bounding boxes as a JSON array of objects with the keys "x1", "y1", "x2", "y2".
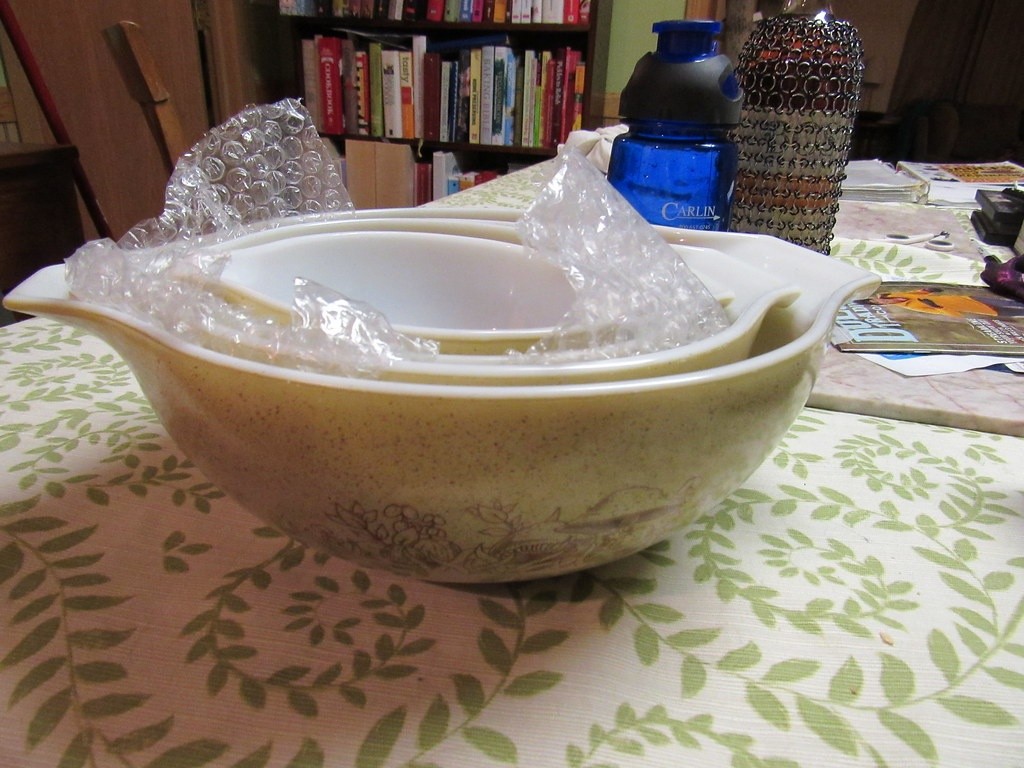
[
  {"x1": 278, "y1": 0, "x2": 590, "y2": 26},
  {"x1": 321, "y1": 138, "x2": 505, "y2": 211},
  {"x1": 301, "y1": 30, "x2": 586, "y2": 150},
  {"x1": 835, "y1": 282, "x2": 1024, "y2": 357}
]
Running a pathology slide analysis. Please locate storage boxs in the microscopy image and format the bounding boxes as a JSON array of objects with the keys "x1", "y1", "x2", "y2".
[{"x1": 345, "y1": 139, "x2": 414, "y2": 209}]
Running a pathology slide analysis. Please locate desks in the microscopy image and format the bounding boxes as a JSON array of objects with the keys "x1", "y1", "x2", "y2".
[{"x1": 0, "y1": 167, "x2": 1023, "y2": 768}]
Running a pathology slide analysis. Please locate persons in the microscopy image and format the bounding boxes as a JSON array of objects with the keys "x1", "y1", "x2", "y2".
[{"x1": 854, "y1": 288, "x2": 1024, "y2": 316}]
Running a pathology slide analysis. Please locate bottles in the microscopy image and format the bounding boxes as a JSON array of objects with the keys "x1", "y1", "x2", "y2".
[
  {"x1": 734, "y1": 0, "x2": 865, "y2": 256},
  {"x1": 607, "y1": 19, "x2": 738, "y2": 232}
]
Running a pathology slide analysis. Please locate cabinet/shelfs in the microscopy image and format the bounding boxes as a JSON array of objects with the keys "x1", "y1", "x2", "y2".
[{"x1": 260, "y1": 0, "x2": 613, "y2": 211}]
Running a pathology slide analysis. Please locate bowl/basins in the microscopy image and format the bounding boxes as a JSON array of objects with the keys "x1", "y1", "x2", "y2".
[{"x1": 2, "y1": 206, "x2": 883, "y2": 584}]
